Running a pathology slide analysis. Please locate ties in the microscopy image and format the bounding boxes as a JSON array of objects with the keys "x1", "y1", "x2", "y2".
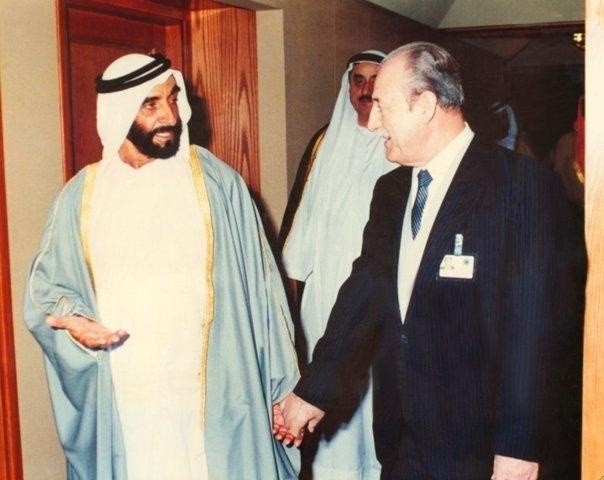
[{"x1": 411, "y1": 169, "x2": 433, "y2": 239}]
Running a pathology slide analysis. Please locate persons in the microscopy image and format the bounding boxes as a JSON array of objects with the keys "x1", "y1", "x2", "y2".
[
  {"x1": 281, "y1": 40, "x2": 556, "y2": 479},
  {"x1": 476, "y1": 96, "x2": 585, "y2": 209},
  {"x1": 275, "y1": 49, "x2": 404, "y2": 480},
  {"x1": 23, "y1": 53, "x2": 303, "y2": 479}
]
